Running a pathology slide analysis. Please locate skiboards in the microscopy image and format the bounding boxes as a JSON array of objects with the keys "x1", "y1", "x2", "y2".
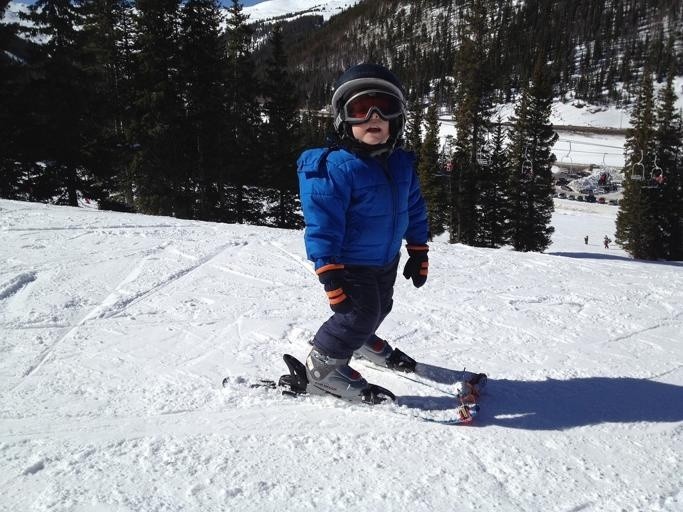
[{"x1": 222, "y1": 329, "x2": 487, "y2": 424}]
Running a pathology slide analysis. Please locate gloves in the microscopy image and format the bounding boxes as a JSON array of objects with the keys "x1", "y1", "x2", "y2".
[
  {"x1": 315, "y1": 264, "x2": 353, "y2": 314},
  {"x1": 402, "y1": 244, "x2": 428, "y2": 288}
]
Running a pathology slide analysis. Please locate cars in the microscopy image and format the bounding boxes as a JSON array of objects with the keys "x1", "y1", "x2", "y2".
[{"x1": 549, "y1": 192, "x2": 616, "y2": 205}]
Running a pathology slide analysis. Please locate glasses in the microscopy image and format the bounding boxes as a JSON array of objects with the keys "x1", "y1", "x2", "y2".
[{"x1": 340, "y1": 88, "x2": 405, "y2": 125}]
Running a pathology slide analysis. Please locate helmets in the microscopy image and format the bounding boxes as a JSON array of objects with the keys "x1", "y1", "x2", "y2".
[{"x1": 330, "y1": 63, "x2": 403, "y2": 113}]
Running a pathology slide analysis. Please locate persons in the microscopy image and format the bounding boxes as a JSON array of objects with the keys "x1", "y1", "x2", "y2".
[
  {"x1": 296, "y1": 64, "x2": 430, "y2": 403},
  {"x1": 603, "y1": 234, "x2": 609, "y2": 247},
  {"x1": 585, "y1": 236, "x2": 588, "y2": 244}
]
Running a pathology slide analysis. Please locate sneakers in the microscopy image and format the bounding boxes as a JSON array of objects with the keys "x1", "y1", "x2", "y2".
[
  {"x1": 353, "y1": 334, "x2": 392, "y2": 359},
  {"x1": 304, "y1": 346, "x2": 368, "y2": 398}
]
